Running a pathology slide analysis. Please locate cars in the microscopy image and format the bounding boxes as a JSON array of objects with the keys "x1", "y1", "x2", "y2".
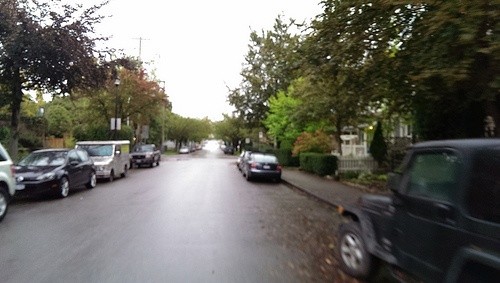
[
  {"x1": 129, "y1": 143, "x2": 161, "y2": 168},
  {"x1": 235, "y1": 149, "x2": 283, "y2": 184},
  {"x1": 179, "y1": 144, "x2": 202, "y2": 154},
  {"x1": 13, "y1": 149, "x2": 97, "y2": 198},
  {"x1": 220, "y1": 145, "x2": 235, "y2": 156}
]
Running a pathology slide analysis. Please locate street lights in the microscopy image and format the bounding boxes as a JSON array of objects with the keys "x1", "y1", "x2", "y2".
[{"x1": 114, "y1": 75, "x2": 122, "y2": 140}]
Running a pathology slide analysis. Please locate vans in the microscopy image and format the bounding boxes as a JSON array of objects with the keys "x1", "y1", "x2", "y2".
[{"x1": 75, "y1": 140, "x2": 130, "y2": 183}]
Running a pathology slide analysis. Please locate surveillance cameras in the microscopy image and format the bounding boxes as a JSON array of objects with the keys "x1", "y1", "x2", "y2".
[{"x1": 114, "y1": 79, "x2": 120, "y2": 86}]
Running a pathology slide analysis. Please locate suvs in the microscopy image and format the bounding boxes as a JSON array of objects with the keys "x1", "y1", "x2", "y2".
[
  {"x1": 0, "y1": 143, "x2": 16, "y2": 222},
  {"x1": 337, "y1": 139, "x2": 500, "y2": 283}
]
